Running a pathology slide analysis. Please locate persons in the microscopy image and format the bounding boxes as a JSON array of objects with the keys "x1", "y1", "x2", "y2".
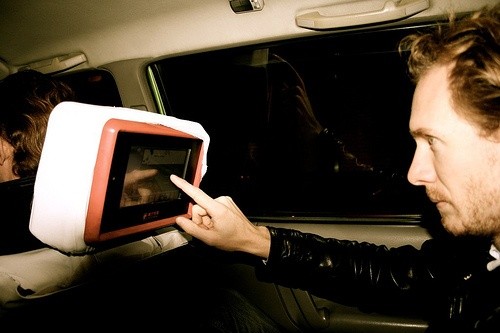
[
  {"x1": 170, "y1": 5, "x2": 500, "y2": 333},
  {"x1": 0, "y1": 69, "x2": 78, "y2": 256},
  {"x1": 271, "y1": 54, "x2": 426, "y2": 206},
  {"x1": 120, "y1": 168, "x2": 166, "y2": 208}
]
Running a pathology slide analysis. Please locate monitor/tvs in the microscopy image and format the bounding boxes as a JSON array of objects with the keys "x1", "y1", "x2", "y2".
[{"x1": 84, "y1": 118, "x2": 204, "y2": 244}]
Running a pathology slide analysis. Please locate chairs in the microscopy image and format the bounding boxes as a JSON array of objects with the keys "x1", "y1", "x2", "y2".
[{"x1": 0, "y1": 229, "x2": 195, "y2": 333}]
